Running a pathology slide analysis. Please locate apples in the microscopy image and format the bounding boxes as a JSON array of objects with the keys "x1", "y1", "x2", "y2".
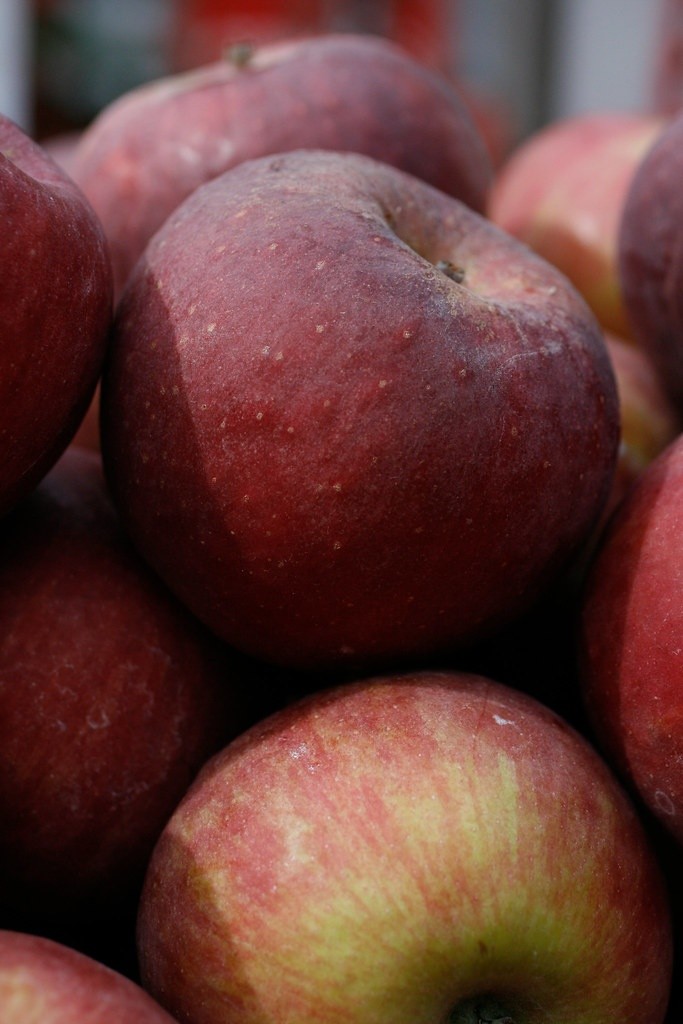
[{"x1": 0, "y1": 0, "x2": 683, "y2": 1024}]
